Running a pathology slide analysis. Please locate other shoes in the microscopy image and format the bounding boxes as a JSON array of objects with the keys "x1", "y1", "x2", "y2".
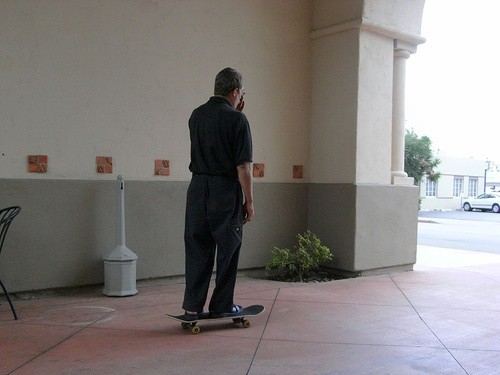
[
  {"x1": 210, "y1": 304, "x2": 243, "y2": 315},
  {"x1": 184, "y1": 310, "x2": 210, "y2": 319}
]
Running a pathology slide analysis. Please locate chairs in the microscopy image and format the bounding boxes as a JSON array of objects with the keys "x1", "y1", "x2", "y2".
[{"x1": 0, "y1": 204, "x2": 21, "y2": 321}]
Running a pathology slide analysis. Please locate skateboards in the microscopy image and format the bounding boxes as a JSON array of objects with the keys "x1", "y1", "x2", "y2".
[{"x1": 165, "y1": 304, "x2": 265, "y2": 333}]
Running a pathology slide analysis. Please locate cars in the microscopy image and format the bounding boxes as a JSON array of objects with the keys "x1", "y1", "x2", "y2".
[{"x1": 461, "y1": 192, "x2": 500, "y2": 214}]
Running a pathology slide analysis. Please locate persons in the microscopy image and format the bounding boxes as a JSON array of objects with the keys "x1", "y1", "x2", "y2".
[{"x1": 181, "y1": 68, "x2": 255, "y2": 320}]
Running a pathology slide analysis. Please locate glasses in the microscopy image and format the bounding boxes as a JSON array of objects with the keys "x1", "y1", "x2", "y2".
[{"x1": 236, "y1": 88, "x2": 245, "y2": 97}]
